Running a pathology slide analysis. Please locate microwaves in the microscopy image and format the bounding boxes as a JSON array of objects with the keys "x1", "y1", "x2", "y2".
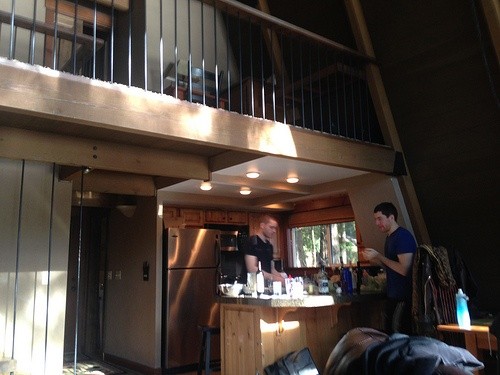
[{"x1": 218, "y1": 230, "x2": 248, "y2": 251}]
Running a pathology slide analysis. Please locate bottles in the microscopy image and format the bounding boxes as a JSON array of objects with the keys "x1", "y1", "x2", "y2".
[
  {"x1": 336, "y1": 281, "x2": 342, "y2": 294},
  {"x1": 257, "y1": 260, "x2": 265, "y2": 295},
  {"x1": 304, "y1": 256, "x2": 345, "y2": 287},
  {"x1": 318, "y1": 265, "x2": 329, "y2": 294},
  {"x1": 307, "y1": 276, "x2": 315, "y2": 295},
  {"x1": 343, "y1": 266, "x2": 353, "y2": 296},
  {"x1": 346, "y1": 261, "x2": 386, "y2": 294},
  {"x1": 342, "y1": 279, "x2": 349, "y2": 296},
  {"x1": 455, "y1": 288, "x2": 471, "y2": 329}
]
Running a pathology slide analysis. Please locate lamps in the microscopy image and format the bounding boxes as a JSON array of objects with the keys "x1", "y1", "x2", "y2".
[{"x1": 200, "y1": 172, "x2": 299, "y2": 195}]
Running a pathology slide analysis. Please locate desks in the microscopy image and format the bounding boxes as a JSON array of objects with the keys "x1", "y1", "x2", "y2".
[{"x1": 437, "y1": 323, "x2": 497, "y2": 375}]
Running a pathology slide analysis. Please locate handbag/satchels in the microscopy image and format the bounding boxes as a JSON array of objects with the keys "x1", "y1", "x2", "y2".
[{"x1": 263, "y1": 347, "x2": 320, "y2": 375}]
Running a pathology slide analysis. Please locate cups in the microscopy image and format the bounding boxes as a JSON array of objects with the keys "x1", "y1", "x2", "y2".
[
  {"x1": 268, "y1": 276, "x2": 275, "y2": 295},
  {"x1": 294, "y1": 277, "x2": 304, "y2": 286},
  {"x1": 273, "y1": 281, "x2": 282, "y2": 296},
  {"x1": 247, "y1": 273, "x2": 256, "y2": 297},
  {"x1": 285, "y1": 278, "x2": 293, "y2": 297},
  {"x1": 292, "y1": 283, "x2": 304, "y2": 297}
]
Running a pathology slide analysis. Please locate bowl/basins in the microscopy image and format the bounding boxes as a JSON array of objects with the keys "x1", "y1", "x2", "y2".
[
  {"x1": 218, "y1": 283, "x2": 243, "y2": 296},
  {"x1": 242, "y1": 284, "x2": 252, "y2": 295}
]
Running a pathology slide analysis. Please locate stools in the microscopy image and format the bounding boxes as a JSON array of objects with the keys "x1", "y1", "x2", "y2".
[{"x1": 197, "y1": 325, "x2": 220, "y2": 375}]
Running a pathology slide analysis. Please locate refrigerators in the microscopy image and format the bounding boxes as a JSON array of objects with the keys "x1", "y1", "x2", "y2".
[{"x1": 161, "y1": 226, "x2": 224, "y2": 375}]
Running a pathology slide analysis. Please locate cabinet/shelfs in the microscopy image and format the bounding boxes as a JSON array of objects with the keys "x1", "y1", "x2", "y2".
[
  {"x1": 163, "y1": 207, "x2": 282, "y2": 259},
  {"x1": 214, "y1": 295, "x2": 386, "y2": 375},
  {"x1": 163, "y1": 76, "x2": 290, "y2": 123}
]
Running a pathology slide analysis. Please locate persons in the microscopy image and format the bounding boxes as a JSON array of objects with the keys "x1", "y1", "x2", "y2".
[
  {"x1": 236, "y1": 212, "x2": 284, "y2": 285},
  {"x1": 362, "y1": 202, "x2": 417, "y2": 337}
]
